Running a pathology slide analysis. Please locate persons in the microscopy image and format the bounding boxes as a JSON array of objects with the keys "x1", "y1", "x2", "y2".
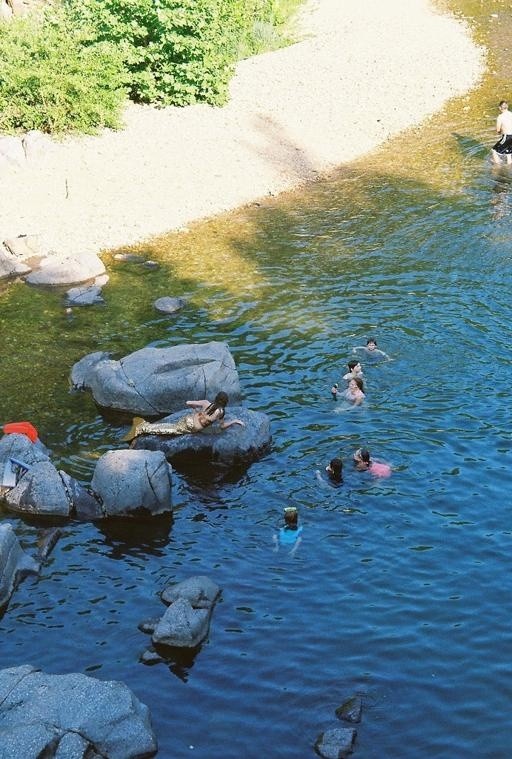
[
  {"x1": 342, "y1": 359, "x2": 367, "y2": 379},
  {"x1": 490, "y1": 100, "x2": 512, "y2": 164},
  {"x1": 272, "y1": 506, "x2": 305, "y2": 559},
  {"x1": 315, "y1": 458, "x2": 345, "y2": 490},
  {"x1": 353, "y1": 338, "x2": 396, "y2": 362},
  {"x1": 352, "y1": 447, "x2": 401, "y2": 480},
  {"x1": 331, "y1": 377, "x2": 366, "y2": 415},
  {"x1": 120, "y1": 391, "x2": 246, "y2": 442}
]
